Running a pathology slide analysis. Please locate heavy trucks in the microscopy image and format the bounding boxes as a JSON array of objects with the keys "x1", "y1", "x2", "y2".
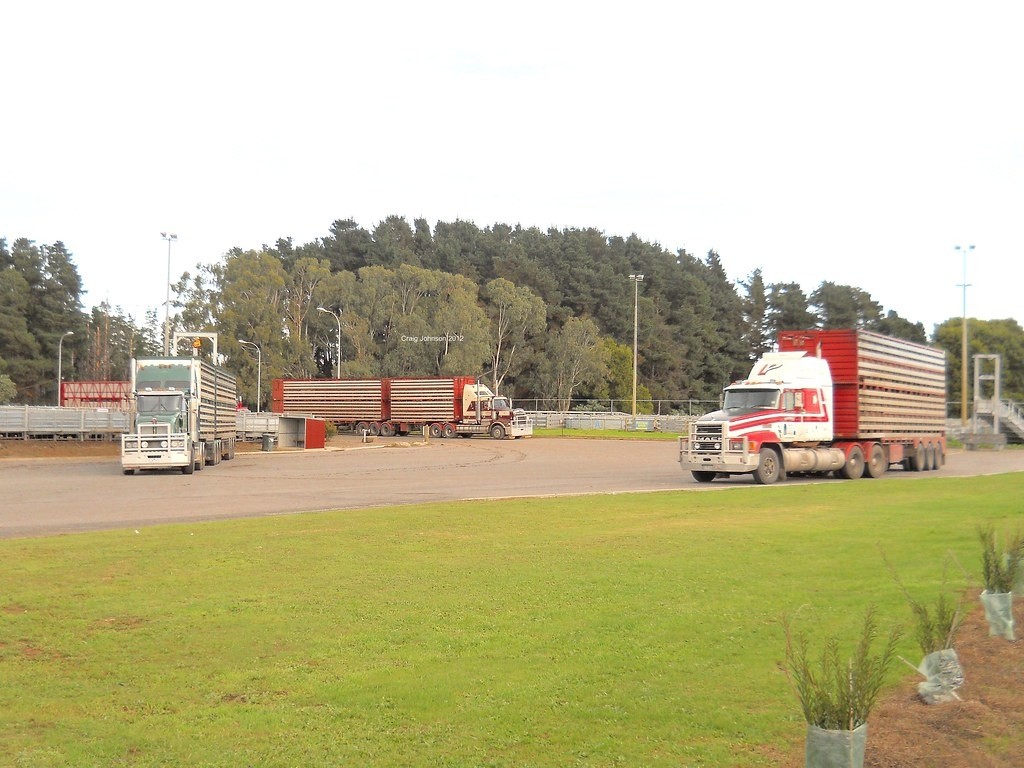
[
  {"x1": 118, "y1": 354, "x2": 238, "y2": 475},
  {"x1": 271, "y1": 377, "x2": 533, "y2": 442},
  {"x1": 677, "y1": 328, "x2": 950, "y2": 486}
]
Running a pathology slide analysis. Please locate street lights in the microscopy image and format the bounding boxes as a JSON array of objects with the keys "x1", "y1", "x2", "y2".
[
  {"x1": 315, "y1": 306, "x2": 341, "y2": 380},
  {"x1": 628, "y1": 274, "x2": 645, "y2": 417},
  {"x1": 160, "y1": 232, "x2": 178, "y2": 358},
  {"x1": 56, "y1": 331, "x2": 74, "y2": 407},
  {"x1": 237, "y1": 339, "x2": 260, "y2": 414}
]
